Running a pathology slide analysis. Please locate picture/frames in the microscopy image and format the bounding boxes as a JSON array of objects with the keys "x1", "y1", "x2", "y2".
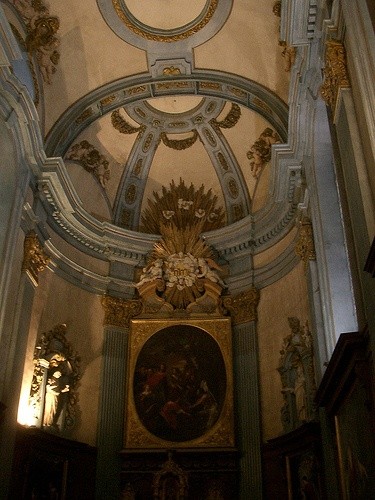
[{"x1": 121, "y1": 317, "x2": 235, "y2": 449}]
